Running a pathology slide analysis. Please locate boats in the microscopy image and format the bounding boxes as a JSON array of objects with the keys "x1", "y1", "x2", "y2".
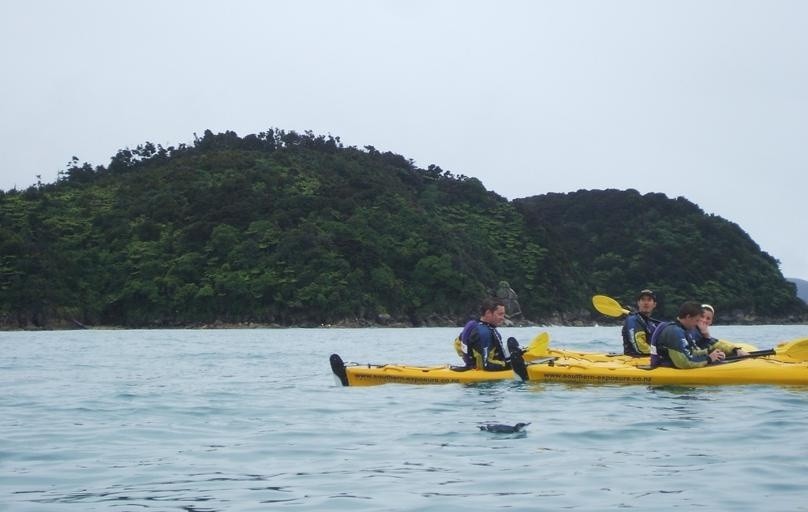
[
  {"x1": 522, "y1": 330, "x2": 808, "y2": 387},
  {"x1": 330, "y1": 331, "x2": 572, "y2": 387}
]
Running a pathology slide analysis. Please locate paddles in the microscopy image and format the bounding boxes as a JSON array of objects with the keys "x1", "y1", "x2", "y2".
[
  {"x1": 507, "y1": 354, "x2": 528, "y2": 380},
  {"x1": 592, "y1": 294, "x2": 664, "y2": 325},
  {"x1": 722, "y1": 338, "x2": 808, "y2": 363},
  {"x1": 508, "y1": 332, "x2": 550, "y2": 358}
]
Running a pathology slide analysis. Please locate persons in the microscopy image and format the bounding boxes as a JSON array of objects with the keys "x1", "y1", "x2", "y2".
[
  {"x1": 455, "y1": 295, "x2": 513, "y2": 371},
  {"x1": 687, "y1": 303, "x2": 750, "y2": 356},
  {"x1": 647, "y1": 299, "x2": 729, "y2": 368},
  {"x1": 621, "y1": 286, "x2": 658, "y2": 357}
]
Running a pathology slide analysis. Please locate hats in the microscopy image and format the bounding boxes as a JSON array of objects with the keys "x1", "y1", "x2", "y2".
[{"x1": 639, "y1": 290, "x2": 656, "y2": 301}]
